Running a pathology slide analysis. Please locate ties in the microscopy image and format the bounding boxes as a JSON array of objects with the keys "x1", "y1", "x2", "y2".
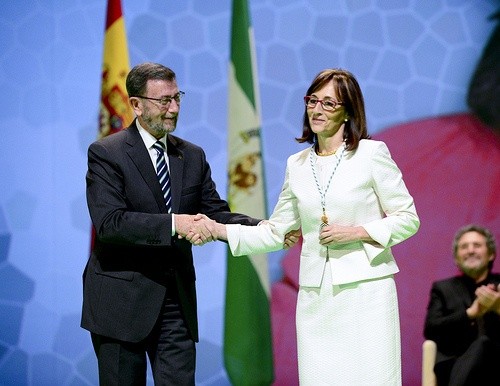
[{"x1": 151, "y1": 140, "x2": 172, "y2": 215}]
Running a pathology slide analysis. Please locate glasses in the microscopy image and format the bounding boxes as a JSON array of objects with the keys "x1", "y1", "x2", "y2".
[
  {"x1": 131, "y1": 91, "x2": 185, "y2": 106},
  {"x1": 303, "y1": 96, "x2": 345, "y2": 112}
]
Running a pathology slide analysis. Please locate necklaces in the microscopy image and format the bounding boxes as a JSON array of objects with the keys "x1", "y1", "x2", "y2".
[{"x1": 310, "y1": 143, "x2": 348, "y2": 233}]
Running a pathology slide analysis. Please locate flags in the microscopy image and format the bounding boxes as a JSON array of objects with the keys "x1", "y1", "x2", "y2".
[
  {"x1": 222, "y1": 0, "x2": 275, "y2": 386},
  {"x1": 90, "y1": 0, "x2": 132, "y2": 252}
]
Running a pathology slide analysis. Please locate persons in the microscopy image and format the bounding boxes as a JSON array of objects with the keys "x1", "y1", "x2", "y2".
[
  {"x1": 178, "y1": 69, "x2": 500, "y2": 386},
  {"x1": 80, "y1": 62, "x2": 302, "y2": 386}
]
